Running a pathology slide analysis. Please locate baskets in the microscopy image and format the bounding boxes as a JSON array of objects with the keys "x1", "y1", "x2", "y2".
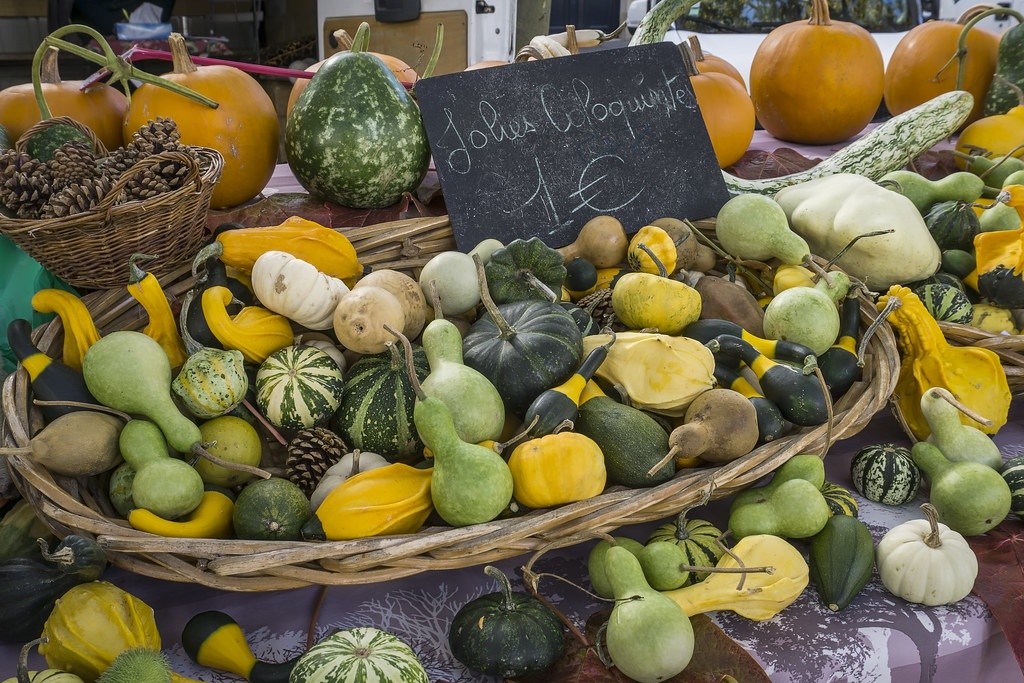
[
  {"x1": 0, "y1": 212, "x2": 901, "y2": 593},
  {"x1": 0, "y1": 116, "x2": 224, "y2": 290},
  {"x1": 892, "y1": 315, "x2": 1024, "y2": 406}
]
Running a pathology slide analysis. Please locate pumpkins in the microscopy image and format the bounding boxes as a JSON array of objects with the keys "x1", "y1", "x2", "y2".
[
  {"x1": 688, "y1": 35, "x2": 749, "y2": 92},
  {"x1": 122, "y1": 32, "x2": 281, "y2": 208},
  {"x1": 3, "y1": 91, "x2": 1023, "y2": 680},
  {"x1": 749, "y1": 0, "x2": 886, "y2": 145},
  {"x1": 627, "y1": 0, "x2": 700, "y2": 47},
  {"x1": 1, "y1": 44, "x2": 131, "y2": 156},
  {"x1": 462, "y1": 60, "x2": 514, "y2": 70},
  {"x1": 286, "y1": 21, "x2": 432, "y2": 207},
  {"x1": 677, "y1": 41, "x2": 756, "y2": 169},
  {"x1": 287, "y1": 28, "x2": 424, "y2": 117},
  {"x1": 884, "y1": 3, "x2": 1003, "y2": 134},
  {"x1": 933, "y1": 8, "x2": 1024, "y2": 116}
]
